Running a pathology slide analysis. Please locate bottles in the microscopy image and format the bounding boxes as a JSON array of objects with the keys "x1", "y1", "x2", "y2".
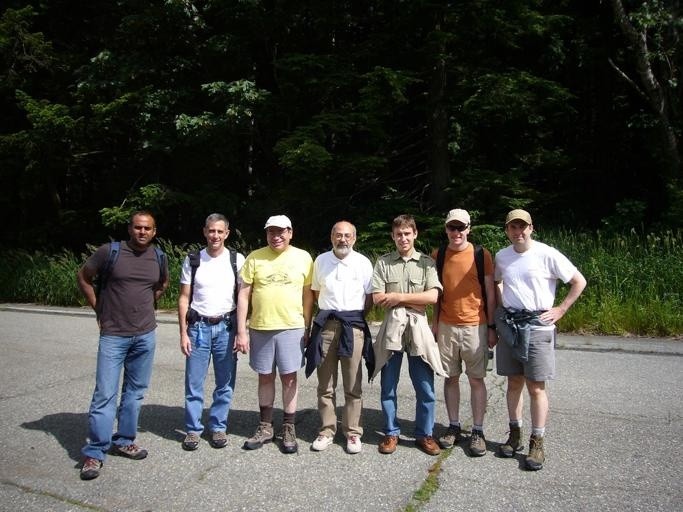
[{"x1": 485, "y1": 347, "x2": 494, "y2": 372}]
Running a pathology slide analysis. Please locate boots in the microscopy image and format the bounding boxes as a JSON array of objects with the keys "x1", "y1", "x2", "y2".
[
  {"x1": 524, "y1": 433, "x2": 545, "y2": 470},
  {"x1": 495, "y1": 425, "x2": 525, "y2": 458}
]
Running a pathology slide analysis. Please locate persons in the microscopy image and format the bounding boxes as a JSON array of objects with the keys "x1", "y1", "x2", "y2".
[
  {"x1": 494, "y1": 208, "x2": 587, "y2": 471},
  {"x1": 177, "y1": 212, "x2": 247, "y2": 451},
  {"x1": 430, "y1": 208, "x2": 497, "y2": 457},
  {"x1": 236, "y1": 215, "x2": 314, "y2": 453},
  {"x1": 369, "y1": 213, "x2": 450, "y2": 456},
  {"x1": 77, "y1": 210, "x2": 169, "y2": 480},
  {"x1": 305, "y1": 220, "x2": 375, "y2": 454}
]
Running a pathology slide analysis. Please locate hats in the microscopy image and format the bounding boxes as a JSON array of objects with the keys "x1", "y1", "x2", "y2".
[
  {"x1": 264, "y1": 215, "x2": 293, "y2": 230},
  {"x1": 505, "y1": 209, "x2": 532, "y2": 225},
  {"x1": 444, "y1": 208, "x2": 470, "y2": 225}
]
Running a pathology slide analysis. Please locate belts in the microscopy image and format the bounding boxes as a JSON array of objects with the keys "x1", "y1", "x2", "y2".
[{"x1": 198, "y1": 311, "x2": 236, "y2": 325}]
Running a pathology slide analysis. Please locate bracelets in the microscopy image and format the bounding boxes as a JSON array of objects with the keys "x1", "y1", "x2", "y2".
[{"x1": 305, "y1": 326, "x2": 310, "y2": 335}]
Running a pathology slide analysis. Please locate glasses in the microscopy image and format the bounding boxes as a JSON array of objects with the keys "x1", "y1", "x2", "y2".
[{"x1": 446, "y1": 223, "x2": 469, "y2": 231}]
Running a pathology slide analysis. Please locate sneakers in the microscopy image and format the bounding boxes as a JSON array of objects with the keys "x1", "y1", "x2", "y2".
[
  {"x1": 182, "y1": 433, "x2": 200, "y2": 450},
  {"x1": 80, "y1": 456, "x2": 104, "y2": 480},
  {"x1": 281, "y1": 423, "x2": 299, "y2": 453},
  {"x1": 211, "y1": 432, "x2": 228, "y2": 448},
  {"x1": 415, "y1": 436, "x2": 440, "y2": 455},
  {"x1": 380, "y1": 435, "x2": 400, "y2": 454},
  {"x1": 312, "y1": 436, "x2": 334, "y2": 451},
  {"x1": 113, "y1": 444, "x2": 148, "y2": 460},
  {"x1": 439, "y1": 423, "x2": 461, "y2": 448},
  {"x1": 347, "y1": 436, "x2": 362, "y2": 454},
  {"x1": 470, "y1": 428, "x2": 487, "y2": 456},
  {"x1": 244, "y1": 421, "x2": 275, "y2": 450}
]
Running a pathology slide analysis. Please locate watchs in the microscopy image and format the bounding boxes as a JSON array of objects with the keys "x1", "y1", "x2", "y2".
[{"x1": 488, "y1": 324, "x2": 496, "y2": 329}]
